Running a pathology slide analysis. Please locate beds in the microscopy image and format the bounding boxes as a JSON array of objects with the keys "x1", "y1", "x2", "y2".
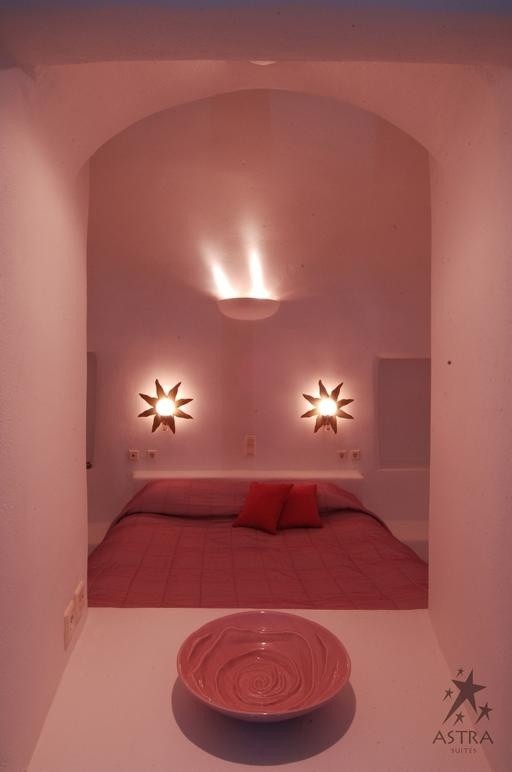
[{"x1": 87, "y1": 476, "x2": 430, "y2": 610}]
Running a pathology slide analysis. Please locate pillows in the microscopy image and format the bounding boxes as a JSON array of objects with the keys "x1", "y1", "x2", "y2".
[
  {"x1": 231, "y1": 479, "x2": 294, "y2": 535},
  {"x1": 279, "y1": 478, "x2": 321, "y2": 530}
]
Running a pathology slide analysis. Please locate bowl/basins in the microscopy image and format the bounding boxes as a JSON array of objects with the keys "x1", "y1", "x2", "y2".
[{"x1": 177, "y1": 610, "x2": 354, "y2": 722}]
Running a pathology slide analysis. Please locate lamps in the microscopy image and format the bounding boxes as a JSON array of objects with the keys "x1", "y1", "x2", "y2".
[
  {"x1": 136, "y1": 378, "x2": 195, "y2": 433},
  {"x1": 302, "y1": 382, "x2": 354, "y2": 434},
  {"x1": 217, "y1": 297, "x2": 280, "y2": 320}
]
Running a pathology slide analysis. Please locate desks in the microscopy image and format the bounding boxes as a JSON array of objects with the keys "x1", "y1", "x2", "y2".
[{"x1": 26, "y1": 602, "x2": 494, "y2": 772}]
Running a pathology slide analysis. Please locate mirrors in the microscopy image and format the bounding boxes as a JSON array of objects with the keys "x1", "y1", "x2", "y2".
[{"x1": 84, "y1": 91, "x2": 433, "y2": 605}]
